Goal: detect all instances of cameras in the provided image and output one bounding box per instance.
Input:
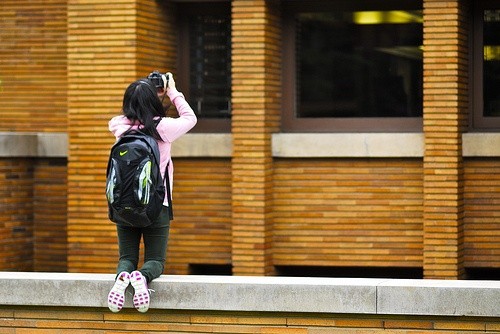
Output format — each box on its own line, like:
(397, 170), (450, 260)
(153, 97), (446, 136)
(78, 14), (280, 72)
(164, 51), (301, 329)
(148, 71), (169, 88)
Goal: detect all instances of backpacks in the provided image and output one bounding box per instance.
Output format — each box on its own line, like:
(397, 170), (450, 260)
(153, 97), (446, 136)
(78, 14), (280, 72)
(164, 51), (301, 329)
(104, 114), (174, 230)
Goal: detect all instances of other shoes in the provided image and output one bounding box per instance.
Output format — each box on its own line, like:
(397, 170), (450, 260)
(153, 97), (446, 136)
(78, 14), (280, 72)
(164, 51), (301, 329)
(129, 270), (155, 313)
(107, 271), (130, 312)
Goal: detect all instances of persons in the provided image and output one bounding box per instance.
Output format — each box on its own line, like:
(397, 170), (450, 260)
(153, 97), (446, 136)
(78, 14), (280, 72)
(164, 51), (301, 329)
(108, 73), (197, 313)
